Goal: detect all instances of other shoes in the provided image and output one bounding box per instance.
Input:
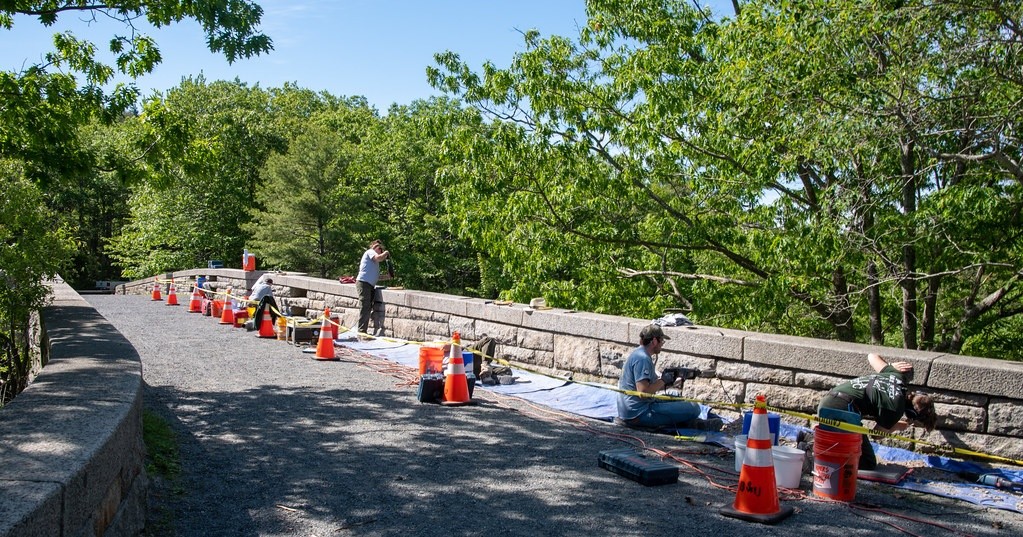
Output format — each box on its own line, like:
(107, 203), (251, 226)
(492, 374), (515, 385)
(358, 335), (369, 341)
(480, 362), (492, 379)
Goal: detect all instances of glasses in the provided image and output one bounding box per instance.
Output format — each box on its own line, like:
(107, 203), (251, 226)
(657, 339), (665, 344)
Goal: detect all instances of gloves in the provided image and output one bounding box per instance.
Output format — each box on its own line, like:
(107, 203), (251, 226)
(662, 367), (679, 386)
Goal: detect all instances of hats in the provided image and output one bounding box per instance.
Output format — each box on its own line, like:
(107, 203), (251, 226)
(639, 324), (671, 340)
(265, 278), (274, 284)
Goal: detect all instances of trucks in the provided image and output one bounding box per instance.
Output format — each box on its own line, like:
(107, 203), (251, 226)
(207, 260), (224, 269)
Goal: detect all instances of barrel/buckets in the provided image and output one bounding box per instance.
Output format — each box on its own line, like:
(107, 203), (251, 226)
(735, 435), (747, 471)
(771, 446), (805, 488)
(420, 347), (444, 374)
(243, 256), (255, 270)
(330, 317), (339, 339)
(812, 425), (862, 501)
(210, 301), (224, 317)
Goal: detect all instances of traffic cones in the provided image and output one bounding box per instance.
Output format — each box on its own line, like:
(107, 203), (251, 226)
(311, 308), (340, 361)
(151, 277), (163, 301)
(164, 277), (180, 306)
(255, 303), (278, 338)
(188, 283), (203, 313)
(719, 396), (795, 525)
(441, 334), (478, 407)
(218, 289), (234, 324)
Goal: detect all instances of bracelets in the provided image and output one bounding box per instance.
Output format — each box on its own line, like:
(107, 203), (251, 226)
(888, 362), (891, 366)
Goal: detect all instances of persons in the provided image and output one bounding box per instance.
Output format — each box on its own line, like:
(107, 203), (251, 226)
(248, 277), (274, 300)
(354, 240), (390, 339)
(796, 353), (938, 472)
(617, 325), (724, 430)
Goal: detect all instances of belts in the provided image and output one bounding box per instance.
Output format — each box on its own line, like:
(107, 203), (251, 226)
(829, 391), (859, 409)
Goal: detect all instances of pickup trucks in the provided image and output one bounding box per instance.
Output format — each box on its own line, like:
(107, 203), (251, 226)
(95, 276), (134, 294)
